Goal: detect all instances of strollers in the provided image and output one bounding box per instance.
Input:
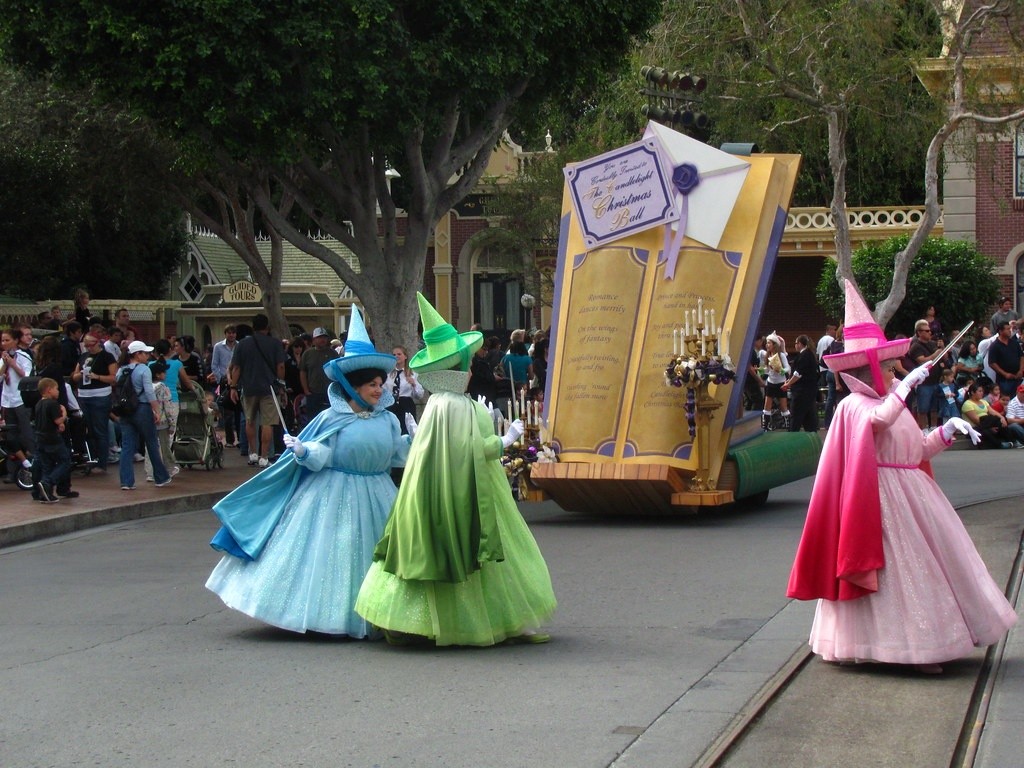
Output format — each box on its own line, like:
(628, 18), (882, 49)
(171, 379), (226, 471)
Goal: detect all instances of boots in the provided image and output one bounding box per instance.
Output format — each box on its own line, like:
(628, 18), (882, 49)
(780, 410), (790, 429)
(761, 409), (775, 433)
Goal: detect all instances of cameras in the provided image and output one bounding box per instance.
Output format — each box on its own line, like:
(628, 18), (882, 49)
(7, 348), (16, 359)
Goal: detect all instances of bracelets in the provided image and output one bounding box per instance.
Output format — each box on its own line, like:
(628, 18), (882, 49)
(230, 385), (238, 389)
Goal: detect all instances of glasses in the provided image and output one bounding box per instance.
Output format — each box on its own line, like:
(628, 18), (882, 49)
(918, 330), (931, 332)
(882, 367), (895, 374)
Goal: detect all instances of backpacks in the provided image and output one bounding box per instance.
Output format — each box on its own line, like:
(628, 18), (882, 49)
(111, 363), (144, 420)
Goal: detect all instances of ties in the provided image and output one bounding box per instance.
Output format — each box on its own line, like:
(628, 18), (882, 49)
(392, 370), (403, 411)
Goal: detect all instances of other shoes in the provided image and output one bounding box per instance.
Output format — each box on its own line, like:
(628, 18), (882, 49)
(1008, 441), (1013, 448)
(1014, 440), (1024, 449)
(914, 664), (943, 674)
(259, 458), (273, 468)
(510, 634), (548, 642)
(248, 453), (259, 465)
(14, 447), (179, 503)
(379, 628), (419, 646)
(225, 443), (235, 448)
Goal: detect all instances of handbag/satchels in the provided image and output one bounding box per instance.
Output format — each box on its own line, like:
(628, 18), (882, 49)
(273, 377), (286, 394)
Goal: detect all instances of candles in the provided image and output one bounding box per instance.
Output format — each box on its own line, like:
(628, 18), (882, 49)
(673, 297), (722, 358)
(477, 387), (538, 446)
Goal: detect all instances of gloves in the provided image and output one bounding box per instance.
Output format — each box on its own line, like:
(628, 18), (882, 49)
(405, 413), (418, 438)
(899, 360), (934, 389)
(942, 417), (982, 445)
(478, 394), (494, 421)
(283, 433), (307, 458)
(502, 419), (525, 448)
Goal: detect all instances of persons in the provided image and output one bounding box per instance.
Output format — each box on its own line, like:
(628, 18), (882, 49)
(204, 304), (417, 640)
(0, 291), (240, 503)
(817, 320), (846, 429)
(226, 313), (375, 468)
(786, 279), (1017, 672)
(743, 331), (821, 432)
(354, 291), (556, 647)
(892, 297), (1024, 448)
(381, 347), (424, 484)
(468, 325), (551, 420)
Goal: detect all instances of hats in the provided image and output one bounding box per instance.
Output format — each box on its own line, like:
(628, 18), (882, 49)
(408, 290), (483, 373)
(1017, 384), (1024, 394)
(322, 303), (397, 383)
(511, 328), (526, 341)
(128, 341), (154, 354)
(330, 339), (344, 356)
(313, 327), (328, 338)
(765, 331), (787, 354)
(823, 278), (911, 372)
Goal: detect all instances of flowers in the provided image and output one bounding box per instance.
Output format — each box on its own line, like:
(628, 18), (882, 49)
(671, 163), (699, 194)
